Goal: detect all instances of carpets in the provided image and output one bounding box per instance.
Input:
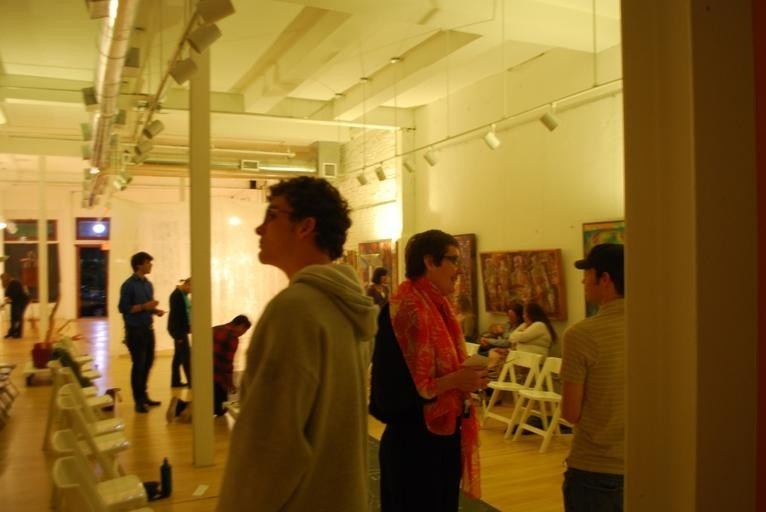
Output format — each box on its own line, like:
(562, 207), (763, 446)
(368, 433), (498, 512)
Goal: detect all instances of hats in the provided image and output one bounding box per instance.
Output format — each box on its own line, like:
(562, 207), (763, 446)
(574, 243), (624, 278)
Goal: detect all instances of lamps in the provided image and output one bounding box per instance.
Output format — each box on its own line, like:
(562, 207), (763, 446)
(353, 101), (562, 189)
(70, 0), (239, 212)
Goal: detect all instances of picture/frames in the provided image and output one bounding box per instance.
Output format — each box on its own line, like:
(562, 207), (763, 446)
(449, 233), (479, 338)
(582, 219), (628, 319)
(478, 248), (567, 322)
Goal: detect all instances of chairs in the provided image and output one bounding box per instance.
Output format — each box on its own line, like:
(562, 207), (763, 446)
(480, 349), (550, 440)
(515, 354), (576, 454)
(463, 341), (489, 418)
(0, 355), (152, 512)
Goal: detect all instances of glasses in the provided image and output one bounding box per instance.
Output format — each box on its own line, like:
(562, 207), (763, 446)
(441, 254), (462, 267)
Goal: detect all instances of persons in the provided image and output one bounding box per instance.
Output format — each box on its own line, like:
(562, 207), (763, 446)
(368, 230), (490, 512)
(0, 272), (31, 339)
(168, 278), (192, 388)
(559, 243), (624, 512)
(369, 268), (389, 310)
(119, 252), (165, 413)
(166, 314), (250, 423)
(214, 176), (381, 511)
(481, 302), (557, 407)
